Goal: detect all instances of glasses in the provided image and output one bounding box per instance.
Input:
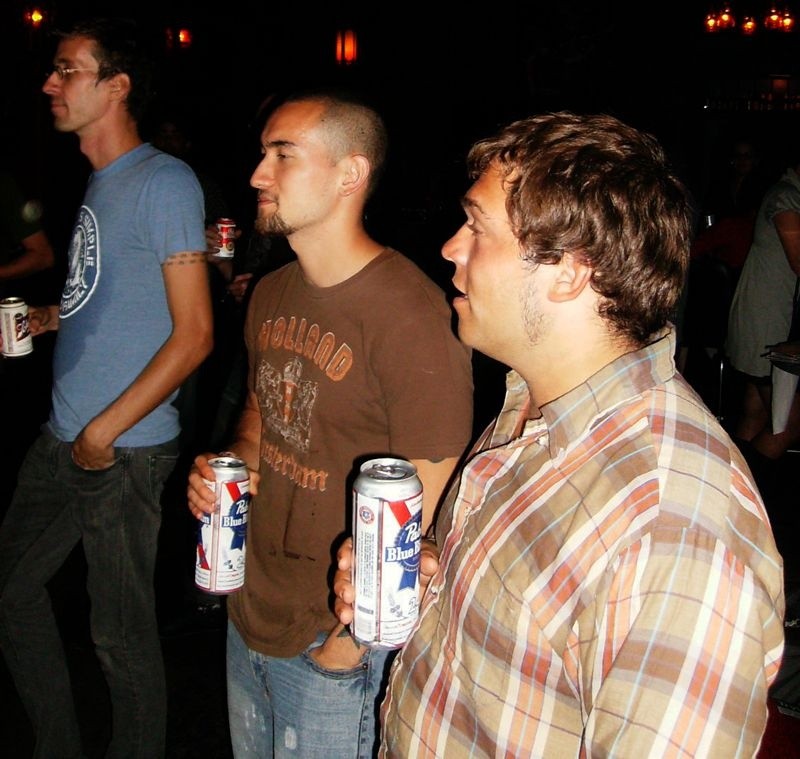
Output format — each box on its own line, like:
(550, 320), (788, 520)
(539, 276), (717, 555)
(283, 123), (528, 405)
(46, 58), (100, 80)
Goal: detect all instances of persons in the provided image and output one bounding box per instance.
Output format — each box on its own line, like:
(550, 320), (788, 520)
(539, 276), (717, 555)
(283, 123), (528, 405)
(187, 97), (474, 757)
(333, 110), (785, 759)
(0, 22), (254, 759)
(725, 151), (800, 490)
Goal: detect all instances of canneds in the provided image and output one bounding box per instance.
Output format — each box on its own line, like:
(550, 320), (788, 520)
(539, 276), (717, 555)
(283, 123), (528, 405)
(212, 218), (237, 259)
(194, 457), (250, 594)
(0, 296), (33, 358)
(350, 458), (427, 649)
(704, 214), (715, 229)
